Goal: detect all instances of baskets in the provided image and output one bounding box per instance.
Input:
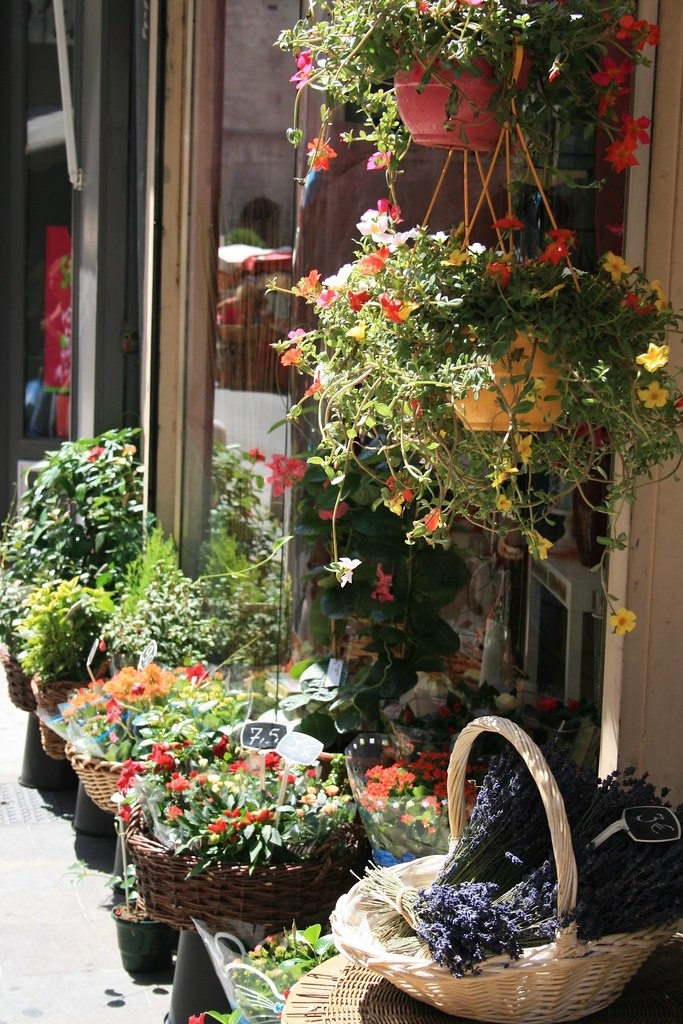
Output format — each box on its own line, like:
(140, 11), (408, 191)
(0, 627), (683, 1024)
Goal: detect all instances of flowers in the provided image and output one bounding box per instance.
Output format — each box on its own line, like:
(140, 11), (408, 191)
(0, 429), (683, 1024)
(273, 0), (661, 261)
(261, 213), (683, 635)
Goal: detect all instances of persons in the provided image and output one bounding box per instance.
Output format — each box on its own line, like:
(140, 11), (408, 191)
(239, 196), (281, 248)
(25, 366), (44, 424)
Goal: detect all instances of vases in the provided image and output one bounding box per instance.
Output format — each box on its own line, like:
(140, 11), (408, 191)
(441, 321), (566, 431)
(111, 835), (135, 892)
(110, 902), (177, 970)
(389, 41), (512, 151)
(163, 927), (234, 1024)
(74, 781), (119, 837)
(18, 713), (81, 792)
